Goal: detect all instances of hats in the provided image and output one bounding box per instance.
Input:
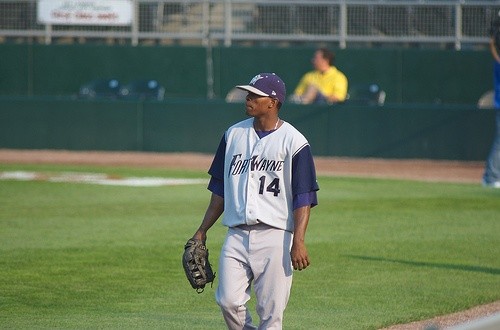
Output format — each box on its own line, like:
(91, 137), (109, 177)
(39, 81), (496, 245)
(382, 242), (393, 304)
(235, 73), (286, 104)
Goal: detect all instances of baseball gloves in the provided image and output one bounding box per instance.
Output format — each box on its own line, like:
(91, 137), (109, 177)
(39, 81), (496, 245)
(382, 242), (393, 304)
(182, 238), (217, 294)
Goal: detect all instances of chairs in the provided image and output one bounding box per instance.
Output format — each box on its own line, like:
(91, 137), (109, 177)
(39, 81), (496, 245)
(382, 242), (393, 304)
(225, 85), (385, 105)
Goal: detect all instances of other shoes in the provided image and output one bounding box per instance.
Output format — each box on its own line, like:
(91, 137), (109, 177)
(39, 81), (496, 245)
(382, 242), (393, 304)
(482, 182), (500, 190)
(289, 94), (301, 105)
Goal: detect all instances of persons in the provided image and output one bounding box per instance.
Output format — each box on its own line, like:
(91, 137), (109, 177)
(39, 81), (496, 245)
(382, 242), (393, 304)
(287, 48), (348, 105)
(182, 72), (318, 330)
(481, 7), (499, 187)
